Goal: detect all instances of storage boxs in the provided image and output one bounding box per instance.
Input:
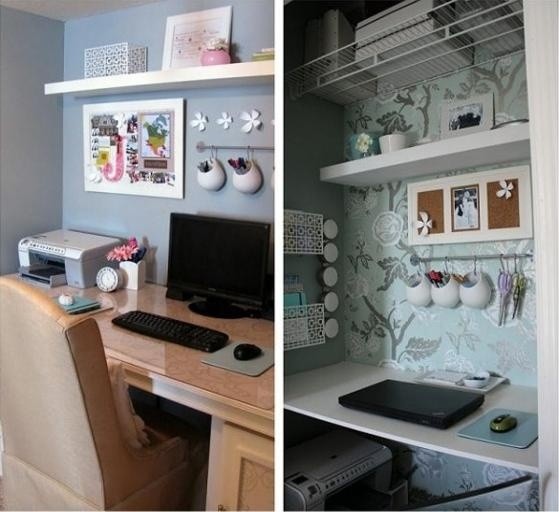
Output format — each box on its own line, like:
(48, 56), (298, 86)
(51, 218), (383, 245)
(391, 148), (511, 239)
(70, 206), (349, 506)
(352, 1), (473, 86)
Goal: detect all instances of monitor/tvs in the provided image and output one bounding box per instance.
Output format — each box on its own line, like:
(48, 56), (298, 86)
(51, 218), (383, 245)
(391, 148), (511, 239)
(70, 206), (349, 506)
(165, 212), (272, 318)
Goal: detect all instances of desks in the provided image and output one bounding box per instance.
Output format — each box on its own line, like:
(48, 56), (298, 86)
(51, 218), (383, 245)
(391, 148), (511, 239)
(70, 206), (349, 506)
(1, 271), (275, 512)
(283, 361), (540, 476)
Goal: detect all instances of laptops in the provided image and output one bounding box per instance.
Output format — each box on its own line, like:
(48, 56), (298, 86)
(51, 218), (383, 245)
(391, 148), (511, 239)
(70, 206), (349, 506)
(337, 379), (486, 430)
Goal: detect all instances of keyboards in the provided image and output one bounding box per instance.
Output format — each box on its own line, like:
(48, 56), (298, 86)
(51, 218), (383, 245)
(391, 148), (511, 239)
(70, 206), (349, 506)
(112, 308), (229, 354)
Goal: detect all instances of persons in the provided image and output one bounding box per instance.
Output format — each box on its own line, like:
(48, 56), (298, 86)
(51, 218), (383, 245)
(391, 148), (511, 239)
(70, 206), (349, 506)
(459, 198), (478, 228)
(455, 189), (477, 226)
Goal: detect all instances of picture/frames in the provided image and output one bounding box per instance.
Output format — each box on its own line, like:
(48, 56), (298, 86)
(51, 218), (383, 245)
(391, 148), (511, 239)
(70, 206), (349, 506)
(440, 93), (494, 139)
(161, 5), (232, 69)
(406, 164), (533, 245)
(81, 97), (186, 199)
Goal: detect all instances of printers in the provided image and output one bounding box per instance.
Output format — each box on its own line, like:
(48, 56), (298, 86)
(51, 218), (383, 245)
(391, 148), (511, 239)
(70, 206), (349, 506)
(17, 227), (119, 290)
(284, 427), (410, 511)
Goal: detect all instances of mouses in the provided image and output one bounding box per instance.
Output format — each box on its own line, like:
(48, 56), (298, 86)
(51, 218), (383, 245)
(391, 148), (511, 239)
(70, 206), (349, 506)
(233, 342), (263, 360)
(488, 413), (518, 432)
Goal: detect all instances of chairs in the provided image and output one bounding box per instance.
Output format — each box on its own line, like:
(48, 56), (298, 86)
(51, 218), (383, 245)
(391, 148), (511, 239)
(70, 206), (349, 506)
(0, 276), (188, 512)
